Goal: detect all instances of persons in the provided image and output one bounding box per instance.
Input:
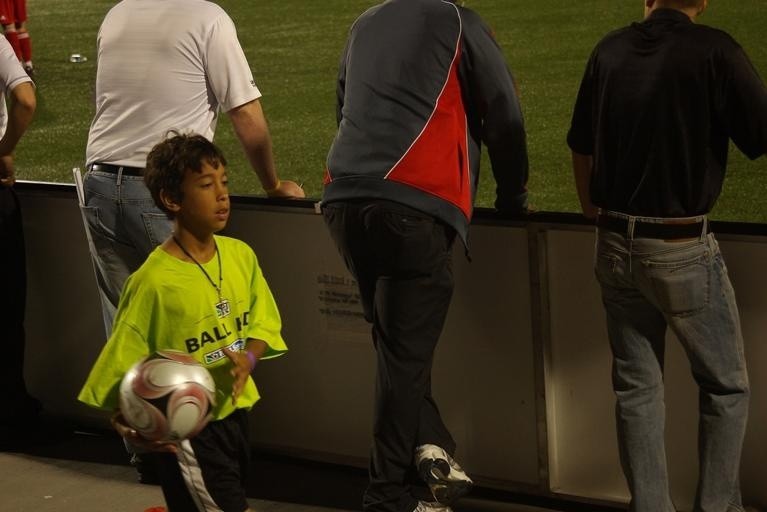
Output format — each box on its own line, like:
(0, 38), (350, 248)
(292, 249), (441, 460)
(565, 1), (767, 512)
(76, 129), (288, 512)
(321, 0), (534, 512)
(0, 0), (33, 73)
(79, 0), (309, 483)
(0, 31), (85, 460)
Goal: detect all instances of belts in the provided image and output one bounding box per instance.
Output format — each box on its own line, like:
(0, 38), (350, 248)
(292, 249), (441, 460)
(595, 213), (710, 240)
(91, 163), (145, 176)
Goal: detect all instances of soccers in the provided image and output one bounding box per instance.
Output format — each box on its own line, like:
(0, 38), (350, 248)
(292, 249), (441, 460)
(120, 348), (216, 442)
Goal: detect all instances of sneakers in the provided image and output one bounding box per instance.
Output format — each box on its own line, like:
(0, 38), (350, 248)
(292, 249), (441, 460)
(413, 498), (453, 512)
(414, 444), (475, 505)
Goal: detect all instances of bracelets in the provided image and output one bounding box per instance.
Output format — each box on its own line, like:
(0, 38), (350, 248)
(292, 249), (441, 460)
(241, 351), (257, 370)
(264, 181), (280, 193)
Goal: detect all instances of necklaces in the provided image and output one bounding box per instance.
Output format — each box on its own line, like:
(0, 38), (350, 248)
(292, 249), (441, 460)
(172, 235), (222, 303)
